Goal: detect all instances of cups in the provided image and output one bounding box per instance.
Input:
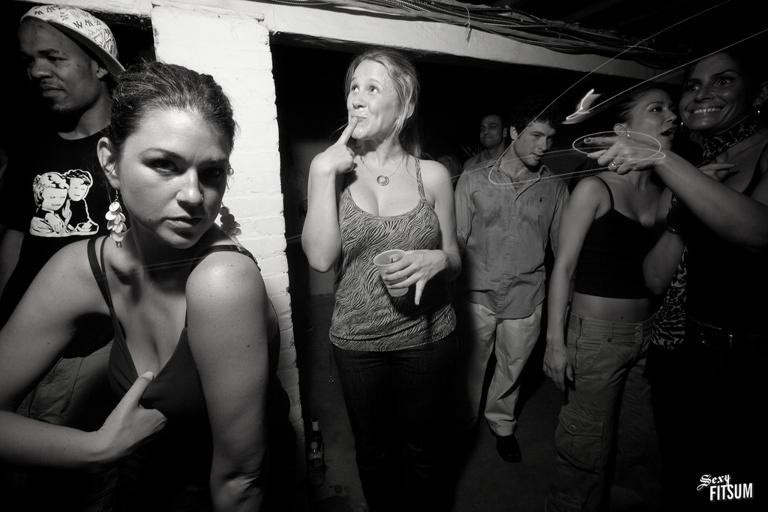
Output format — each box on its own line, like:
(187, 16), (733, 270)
(372, 249), (409, 298)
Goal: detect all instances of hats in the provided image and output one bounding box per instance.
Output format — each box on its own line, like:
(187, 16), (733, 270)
(21, 5), (126, 77)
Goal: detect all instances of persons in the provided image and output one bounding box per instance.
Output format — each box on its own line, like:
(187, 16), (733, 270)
(300, 45), (768, 512)
(29, 169), (100, 237)
(0, 5), (129, 428)
(0, 62), (311, 512)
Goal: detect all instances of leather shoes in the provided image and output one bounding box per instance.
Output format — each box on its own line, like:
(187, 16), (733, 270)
(489, 426), (520, 463)
